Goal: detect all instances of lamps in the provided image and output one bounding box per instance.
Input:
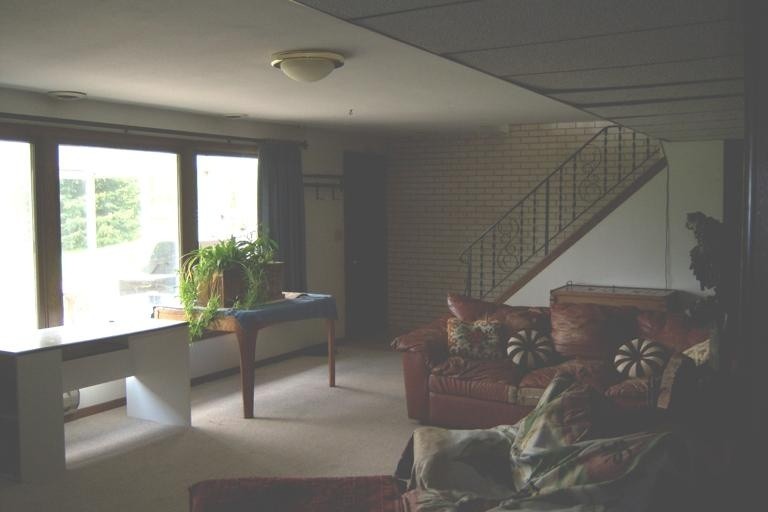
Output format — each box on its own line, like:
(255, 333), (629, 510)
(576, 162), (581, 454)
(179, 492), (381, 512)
(270, 48), (343, 85)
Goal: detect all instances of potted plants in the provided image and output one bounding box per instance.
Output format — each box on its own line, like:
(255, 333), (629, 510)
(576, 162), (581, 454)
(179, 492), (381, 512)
(179, 236), (282, 344)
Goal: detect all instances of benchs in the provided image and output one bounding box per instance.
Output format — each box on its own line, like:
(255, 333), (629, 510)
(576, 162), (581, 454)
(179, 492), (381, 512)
(188, 476), (405, 511)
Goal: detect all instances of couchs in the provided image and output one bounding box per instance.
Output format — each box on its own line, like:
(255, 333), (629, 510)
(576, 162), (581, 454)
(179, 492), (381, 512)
(390, 292), (723, 426)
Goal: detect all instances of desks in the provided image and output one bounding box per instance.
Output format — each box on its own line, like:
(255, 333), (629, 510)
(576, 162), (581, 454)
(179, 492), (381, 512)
(151, 292), (338, 419)
(0, 314), (191, 484)
(551, 286), (676, 309)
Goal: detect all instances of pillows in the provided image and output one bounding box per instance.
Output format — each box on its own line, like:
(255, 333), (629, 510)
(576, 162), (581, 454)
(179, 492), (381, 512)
(446, 311), (711, 408)
